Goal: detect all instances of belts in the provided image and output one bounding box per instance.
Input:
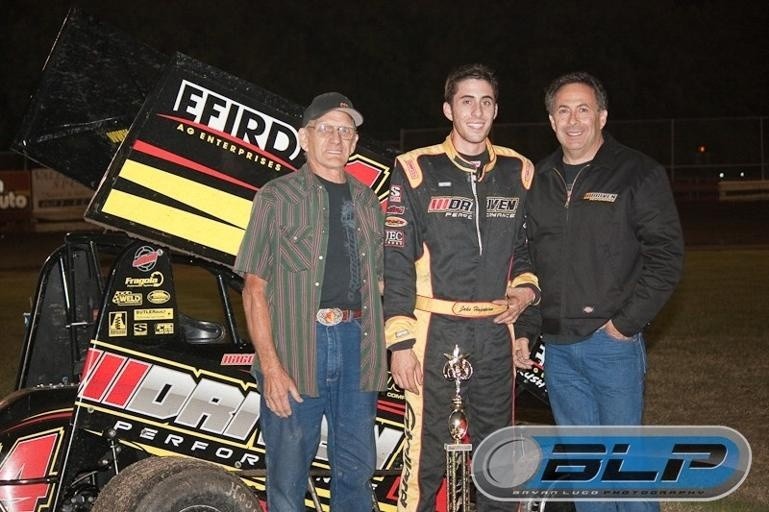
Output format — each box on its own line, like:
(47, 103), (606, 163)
(316, 306), (361, 328)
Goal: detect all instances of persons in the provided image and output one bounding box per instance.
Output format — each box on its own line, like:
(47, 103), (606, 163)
(511, 70), (685, 511)
(233, 92), (389, 512)
(382, 64), (541, 512)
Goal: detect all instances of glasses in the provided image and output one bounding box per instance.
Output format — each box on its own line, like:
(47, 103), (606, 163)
(305, 123), (356, 139)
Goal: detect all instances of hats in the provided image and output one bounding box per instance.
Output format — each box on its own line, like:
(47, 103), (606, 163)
(301, 90), (364, 129)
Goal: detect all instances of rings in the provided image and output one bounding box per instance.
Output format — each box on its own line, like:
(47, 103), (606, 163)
(507, 299), (511, 306)
